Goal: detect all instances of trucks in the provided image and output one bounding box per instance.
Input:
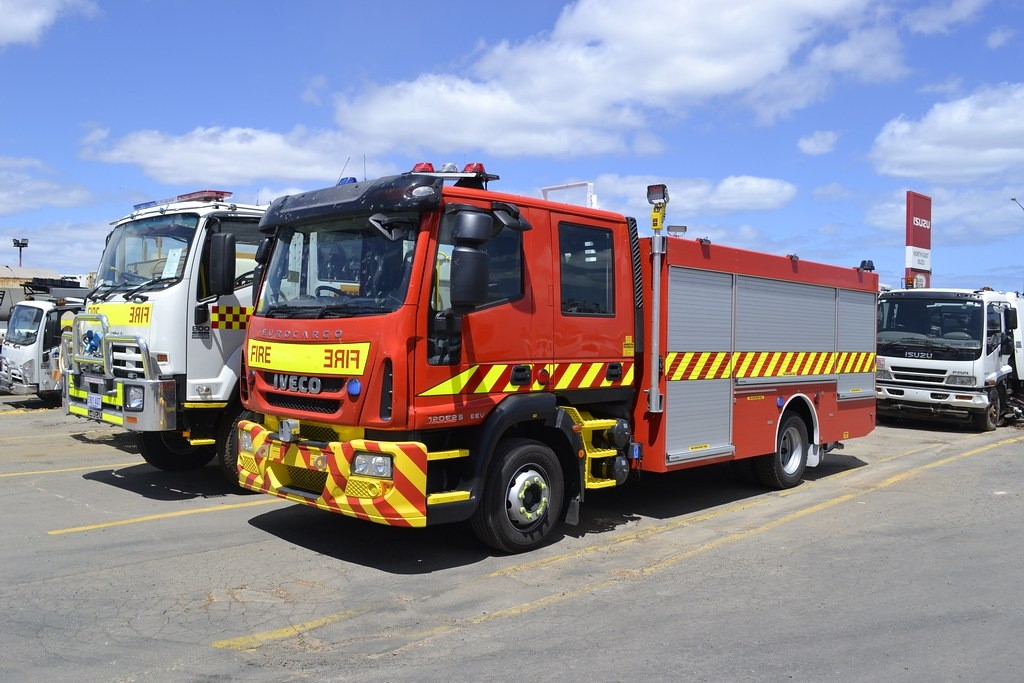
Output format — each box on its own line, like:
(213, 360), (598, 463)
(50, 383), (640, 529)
(875, 287), (1024, 432)
(0, 294), (88, 410)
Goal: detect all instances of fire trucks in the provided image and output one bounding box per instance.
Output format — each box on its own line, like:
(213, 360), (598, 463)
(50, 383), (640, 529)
(236, 161), (878, 552)
(62, 190), (457, 489)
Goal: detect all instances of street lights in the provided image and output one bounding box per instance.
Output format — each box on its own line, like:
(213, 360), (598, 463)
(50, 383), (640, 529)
(13, 238), (29, 267)
